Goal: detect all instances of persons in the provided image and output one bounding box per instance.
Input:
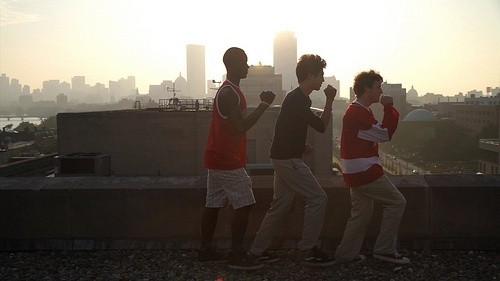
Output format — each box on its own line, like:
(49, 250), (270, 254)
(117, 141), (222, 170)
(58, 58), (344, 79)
(246, 52), (338, 267)
(198, 46), (277, 272)
(333, 70), (411, 265)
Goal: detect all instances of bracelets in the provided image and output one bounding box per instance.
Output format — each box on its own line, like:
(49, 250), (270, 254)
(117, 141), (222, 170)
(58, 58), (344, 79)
(324, 106), (332, 111)
(261, 100), (270, 105)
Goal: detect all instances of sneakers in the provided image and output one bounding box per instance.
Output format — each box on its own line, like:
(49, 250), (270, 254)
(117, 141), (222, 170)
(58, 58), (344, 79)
(373, 250), (411, 264)
(336, 254), (367, 265)
(227, 253), (264, 270)
(246, 252), (281, 263)
(196, 245), (229, 263)
(303, 248), (337, 266)
